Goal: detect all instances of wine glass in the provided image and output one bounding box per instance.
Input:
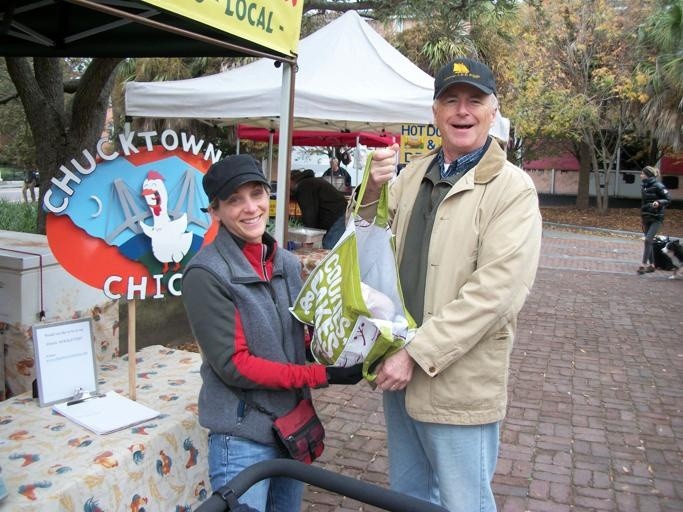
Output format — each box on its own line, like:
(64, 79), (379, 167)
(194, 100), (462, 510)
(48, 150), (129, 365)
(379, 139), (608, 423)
(392, 382), (400, 389)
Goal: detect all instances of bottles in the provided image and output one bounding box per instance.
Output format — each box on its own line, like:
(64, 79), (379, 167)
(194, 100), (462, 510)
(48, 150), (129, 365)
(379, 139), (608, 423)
(642, 165), (656, 177)
(202, 153), (272, 203)
(435, 58), (495, 100)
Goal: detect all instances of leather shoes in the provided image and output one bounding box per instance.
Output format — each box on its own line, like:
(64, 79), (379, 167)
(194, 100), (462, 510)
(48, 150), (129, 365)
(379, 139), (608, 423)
(326, 359), (375, 385)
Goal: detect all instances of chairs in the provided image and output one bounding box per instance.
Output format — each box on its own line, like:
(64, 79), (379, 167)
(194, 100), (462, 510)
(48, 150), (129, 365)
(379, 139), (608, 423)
(637, 266), (656, 273)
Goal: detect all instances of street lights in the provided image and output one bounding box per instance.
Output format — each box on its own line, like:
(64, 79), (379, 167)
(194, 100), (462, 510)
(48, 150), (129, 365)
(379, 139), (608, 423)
(289, 152), (418, 380)
(272, 398), (325, 465)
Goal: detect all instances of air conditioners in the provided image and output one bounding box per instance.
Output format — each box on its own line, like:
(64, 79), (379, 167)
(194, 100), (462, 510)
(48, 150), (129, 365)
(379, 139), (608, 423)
(660, 233), (683, 282)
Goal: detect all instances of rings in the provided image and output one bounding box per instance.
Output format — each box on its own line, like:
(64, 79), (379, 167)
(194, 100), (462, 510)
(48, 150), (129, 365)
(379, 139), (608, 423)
(67, 394), (106, 406)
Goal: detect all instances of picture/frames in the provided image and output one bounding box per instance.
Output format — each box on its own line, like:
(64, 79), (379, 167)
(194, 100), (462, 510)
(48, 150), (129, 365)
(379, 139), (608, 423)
(0, 345), (212, 511)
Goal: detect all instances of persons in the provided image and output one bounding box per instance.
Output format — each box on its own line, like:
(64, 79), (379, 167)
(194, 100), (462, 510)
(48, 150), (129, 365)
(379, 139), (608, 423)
(351, 55), (543, 510)
(320, 156), (351, 187)
(289, 169), (348, 250)
(635, 165), (673, 274)
(178, 151), (385, 510)
